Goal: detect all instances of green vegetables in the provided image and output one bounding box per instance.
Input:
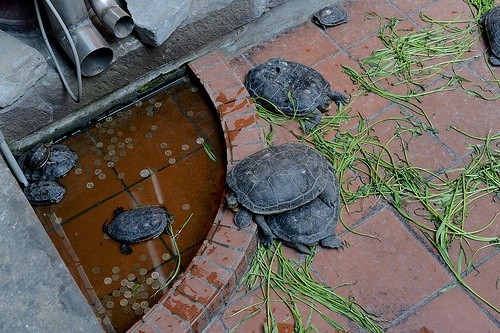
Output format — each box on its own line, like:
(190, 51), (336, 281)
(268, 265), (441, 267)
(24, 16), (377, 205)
(226, 0), (500, 332)
